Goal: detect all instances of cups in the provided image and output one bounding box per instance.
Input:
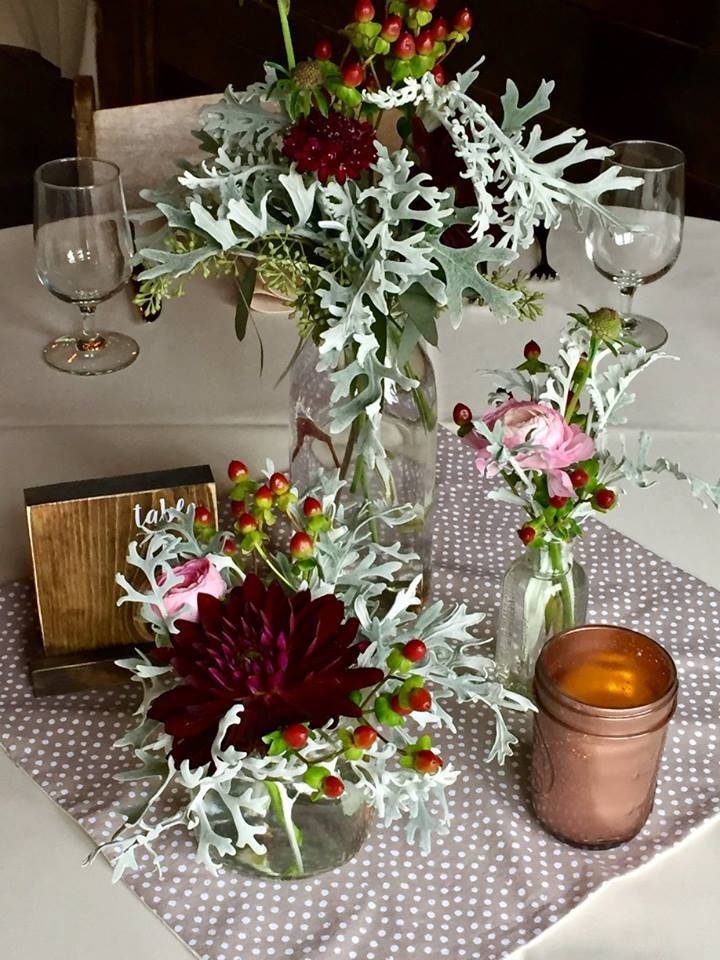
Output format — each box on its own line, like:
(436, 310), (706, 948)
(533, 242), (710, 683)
(530, 624), (679, 851)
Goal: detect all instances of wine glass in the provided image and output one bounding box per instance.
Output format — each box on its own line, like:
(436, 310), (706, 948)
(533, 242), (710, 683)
(584, 141), (684, 353)
(33, 156), (140, 376)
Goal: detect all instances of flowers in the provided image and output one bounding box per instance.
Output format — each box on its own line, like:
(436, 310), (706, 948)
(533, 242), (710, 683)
(82, 460), (539, 882)
(450, 308), (719, 547)
(128, 0), (644, 468)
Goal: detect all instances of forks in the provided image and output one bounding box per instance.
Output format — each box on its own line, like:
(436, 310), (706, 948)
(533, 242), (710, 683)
(530, 219), (558, 280)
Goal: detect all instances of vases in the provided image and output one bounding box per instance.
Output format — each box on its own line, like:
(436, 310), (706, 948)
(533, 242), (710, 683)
(492, 530), (590, 687)
(190, 767), (373, 878)
(290, 319), (436, 605)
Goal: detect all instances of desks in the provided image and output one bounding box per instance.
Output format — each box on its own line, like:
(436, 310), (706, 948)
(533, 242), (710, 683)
(0, 202), (720, 960)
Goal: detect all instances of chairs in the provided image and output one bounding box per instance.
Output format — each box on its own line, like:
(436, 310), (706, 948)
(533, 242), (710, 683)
(72, 86), (297, 214)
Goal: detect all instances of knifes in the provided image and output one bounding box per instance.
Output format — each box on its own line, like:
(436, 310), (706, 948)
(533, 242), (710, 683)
(129, 219), (163, 322)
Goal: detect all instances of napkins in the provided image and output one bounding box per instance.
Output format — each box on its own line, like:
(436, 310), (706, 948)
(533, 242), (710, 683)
(235, 258), (298, 317)
